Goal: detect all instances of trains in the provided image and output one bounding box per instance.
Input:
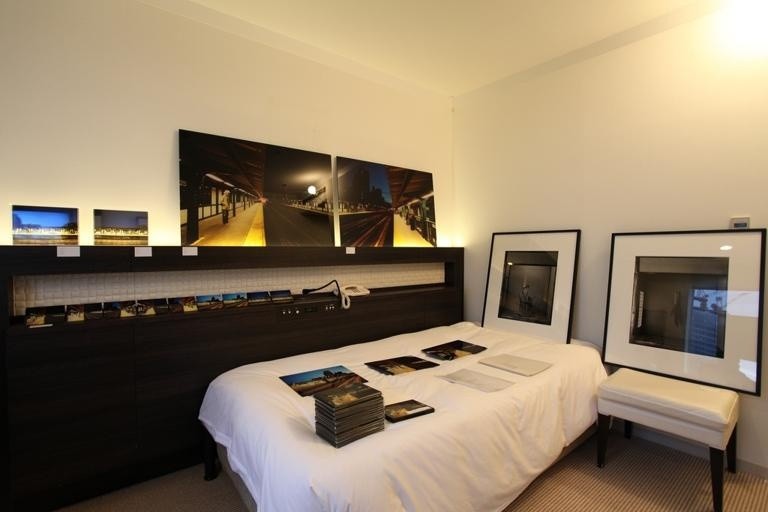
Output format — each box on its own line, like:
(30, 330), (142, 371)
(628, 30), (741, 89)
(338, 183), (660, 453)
(397, 191), (437, 248)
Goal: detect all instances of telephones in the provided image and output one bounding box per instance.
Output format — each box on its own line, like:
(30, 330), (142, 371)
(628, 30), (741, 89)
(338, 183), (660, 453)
(333, 284), (370, 297)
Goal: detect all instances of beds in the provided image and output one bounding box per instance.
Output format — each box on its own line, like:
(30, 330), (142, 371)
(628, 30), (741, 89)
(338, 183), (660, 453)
(197, 320), (608, 512)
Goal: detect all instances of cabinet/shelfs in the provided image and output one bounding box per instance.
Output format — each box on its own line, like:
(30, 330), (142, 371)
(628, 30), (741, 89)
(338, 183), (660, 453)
(0, 246), (465, 512)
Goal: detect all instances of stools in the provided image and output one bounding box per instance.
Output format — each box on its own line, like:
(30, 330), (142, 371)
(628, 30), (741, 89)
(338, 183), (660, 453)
(597, 367), (740, 512)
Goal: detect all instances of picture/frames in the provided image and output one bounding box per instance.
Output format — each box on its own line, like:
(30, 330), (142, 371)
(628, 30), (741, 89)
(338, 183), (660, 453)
(481, 231), (585, 345)
(602, 228), (768, 397)
